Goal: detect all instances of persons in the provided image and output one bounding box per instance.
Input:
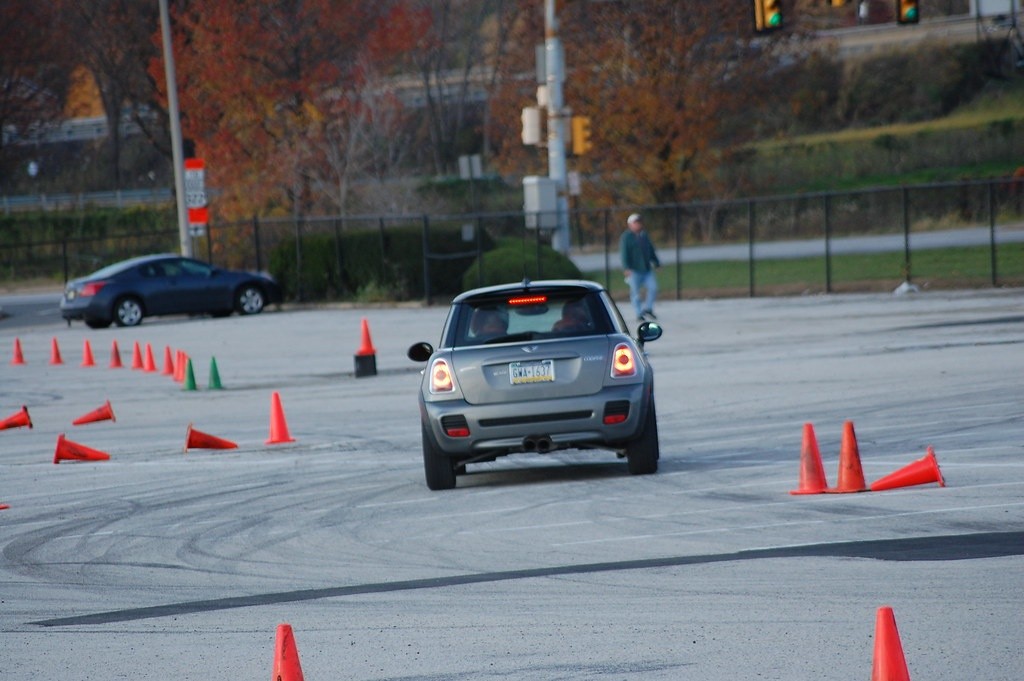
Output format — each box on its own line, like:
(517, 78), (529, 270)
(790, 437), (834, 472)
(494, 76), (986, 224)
(471, 307), (509, 335)
(562, 303), (589, 320)
(620, 213), (661, 321)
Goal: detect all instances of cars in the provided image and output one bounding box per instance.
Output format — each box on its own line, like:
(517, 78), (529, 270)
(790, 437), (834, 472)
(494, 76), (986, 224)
(58, 254), (283, 329)
(406, 278), (666, 490)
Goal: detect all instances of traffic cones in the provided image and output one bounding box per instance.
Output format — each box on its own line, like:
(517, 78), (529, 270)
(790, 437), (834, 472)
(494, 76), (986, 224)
(263, 392), (296, 446)
(788, 422), (829, 494)
(10, 335), (25, 366)
(53, 433), (110, 463)
(272, 624), (304, 681)
(181, 358), (201, 392)
(183, 423), (238, 452)
(71, 401), (117, 425)
(356, 319), (378, 356)
(820, 418), (869, 493)
(49, 338), (188, 383)
(869, 445), (946, 489)
(203, 356), (227, 390)
(871, 606), (912, 681)
(0, 404), (34, 432)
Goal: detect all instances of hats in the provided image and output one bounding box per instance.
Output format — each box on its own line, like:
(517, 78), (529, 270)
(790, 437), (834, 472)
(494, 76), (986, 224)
(627, 214), (643, 223)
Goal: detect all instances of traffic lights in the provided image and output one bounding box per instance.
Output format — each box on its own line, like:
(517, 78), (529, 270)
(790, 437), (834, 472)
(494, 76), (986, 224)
(755, 1), (781, 33)
(575, 115), (594, 156)
(900, 0), (917, 20)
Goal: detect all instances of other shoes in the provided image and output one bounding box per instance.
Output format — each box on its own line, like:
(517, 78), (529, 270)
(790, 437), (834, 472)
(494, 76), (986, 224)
(636, 316), (646, 323)
(642, 309), (656, 319)
